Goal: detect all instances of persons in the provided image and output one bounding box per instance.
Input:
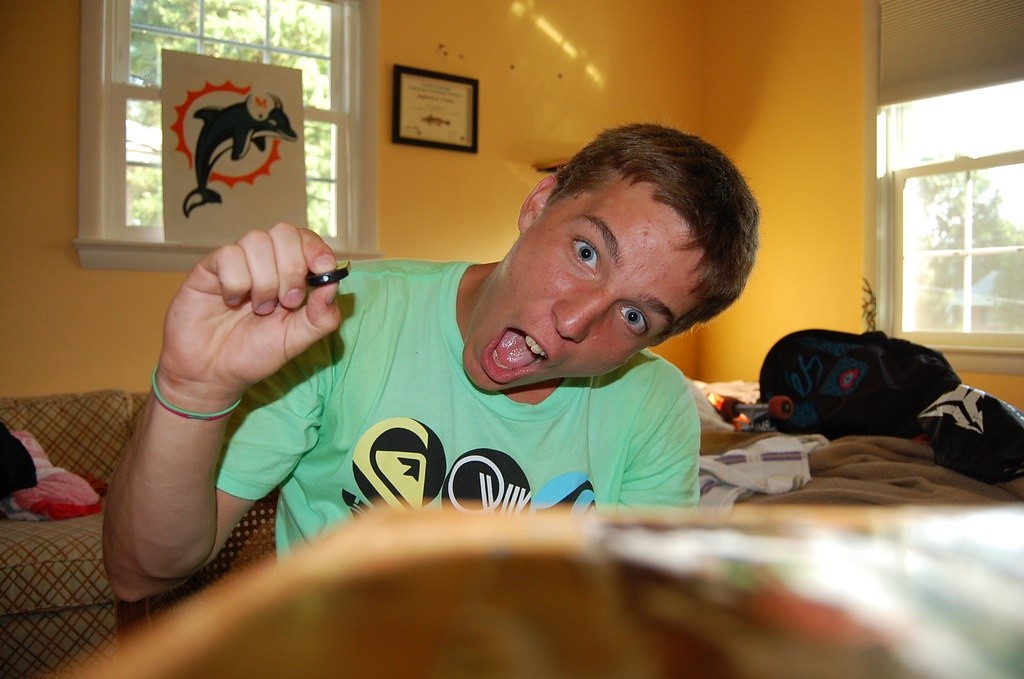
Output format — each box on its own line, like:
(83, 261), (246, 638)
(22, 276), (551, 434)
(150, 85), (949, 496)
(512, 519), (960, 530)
(100, 122), (758, 603)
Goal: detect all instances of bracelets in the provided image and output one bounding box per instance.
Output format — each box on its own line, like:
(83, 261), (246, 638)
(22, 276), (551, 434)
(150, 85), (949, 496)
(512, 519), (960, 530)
(152, 363), (242, 421)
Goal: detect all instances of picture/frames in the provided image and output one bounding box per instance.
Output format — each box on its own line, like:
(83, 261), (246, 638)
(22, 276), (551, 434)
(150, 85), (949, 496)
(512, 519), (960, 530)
(392, 64), (479, 154)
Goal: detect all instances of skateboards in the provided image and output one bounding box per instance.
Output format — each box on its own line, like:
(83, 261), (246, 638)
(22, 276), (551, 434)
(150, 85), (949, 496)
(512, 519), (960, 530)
(699, 396), (796, 456)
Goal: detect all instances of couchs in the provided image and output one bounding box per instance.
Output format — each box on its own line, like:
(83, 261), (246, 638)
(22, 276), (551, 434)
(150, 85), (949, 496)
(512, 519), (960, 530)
(0, 390), (151, 679)
